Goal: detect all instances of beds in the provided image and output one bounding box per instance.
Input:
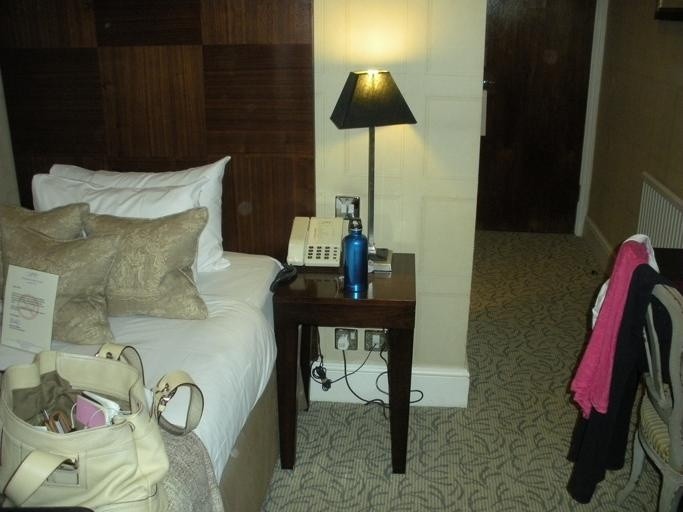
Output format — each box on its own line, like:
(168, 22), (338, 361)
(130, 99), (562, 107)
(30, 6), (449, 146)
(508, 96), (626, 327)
(0, 250), (308, 511)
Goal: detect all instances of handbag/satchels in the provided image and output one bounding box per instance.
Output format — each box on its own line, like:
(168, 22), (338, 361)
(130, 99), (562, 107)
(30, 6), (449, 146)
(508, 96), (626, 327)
(0, 339), (206, 510)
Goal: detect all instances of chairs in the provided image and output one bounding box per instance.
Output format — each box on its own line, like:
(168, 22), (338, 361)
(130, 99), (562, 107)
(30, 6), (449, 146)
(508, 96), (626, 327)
(607, 283), (683, 511)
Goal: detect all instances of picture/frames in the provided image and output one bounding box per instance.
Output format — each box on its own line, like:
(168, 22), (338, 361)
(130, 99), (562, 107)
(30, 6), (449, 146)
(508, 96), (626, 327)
(652, 0), (683, 23)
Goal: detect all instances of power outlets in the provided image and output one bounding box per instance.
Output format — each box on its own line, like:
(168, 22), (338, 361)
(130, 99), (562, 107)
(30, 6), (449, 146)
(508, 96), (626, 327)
(363, 329), (387, 352)
(334, 328), (358, 351)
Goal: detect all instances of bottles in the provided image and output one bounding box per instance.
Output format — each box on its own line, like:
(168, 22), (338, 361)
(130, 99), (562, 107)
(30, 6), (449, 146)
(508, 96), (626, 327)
(343, 219), (368, 291)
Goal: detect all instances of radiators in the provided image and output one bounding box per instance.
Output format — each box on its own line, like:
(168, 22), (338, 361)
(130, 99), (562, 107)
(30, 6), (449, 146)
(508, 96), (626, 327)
(636, 168), (683, 250)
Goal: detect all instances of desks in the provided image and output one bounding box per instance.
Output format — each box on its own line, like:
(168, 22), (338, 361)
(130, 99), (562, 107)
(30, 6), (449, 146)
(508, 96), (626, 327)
(269, 250), (419, 468)
(649, 245), (683, 381)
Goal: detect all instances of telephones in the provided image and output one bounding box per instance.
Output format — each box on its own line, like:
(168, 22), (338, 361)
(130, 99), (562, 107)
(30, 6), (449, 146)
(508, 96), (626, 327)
(286, 215), (344, 268)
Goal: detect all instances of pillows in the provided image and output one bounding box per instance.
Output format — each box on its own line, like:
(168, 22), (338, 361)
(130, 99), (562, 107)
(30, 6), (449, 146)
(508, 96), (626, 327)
(0, 196), (88, 242)
(77, 201), (210, 323)
(0, 219), (124, 347)
(27, 171), (207, 281)
(47, 151), (234, 274)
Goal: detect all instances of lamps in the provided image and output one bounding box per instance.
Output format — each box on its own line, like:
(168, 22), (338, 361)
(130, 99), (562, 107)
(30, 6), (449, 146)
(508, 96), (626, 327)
(327, 69), (418, 273)
